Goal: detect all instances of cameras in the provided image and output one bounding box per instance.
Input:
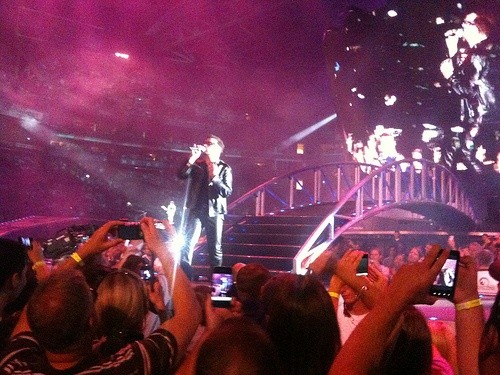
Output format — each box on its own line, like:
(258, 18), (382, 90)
(140, 266), (155, 282)
(18, 236), (33, 249)
(117, 222), (144, 240)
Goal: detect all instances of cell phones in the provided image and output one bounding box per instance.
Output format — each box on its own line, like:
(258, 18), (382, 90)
(430, 249), (460, 299)
(356, 253), (369, 276)
(212, 267), (234, 307)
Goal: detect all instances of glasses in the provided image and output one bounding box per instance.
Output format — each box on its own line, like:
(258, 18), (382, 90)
(462, 19), (475, 26)
(203, 142), (216, 146)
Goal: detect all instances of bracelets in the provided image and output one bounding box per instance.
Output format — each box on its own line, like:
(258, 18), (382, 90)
(187, 160), (192, 166)
(70, 253), (86, 267)
(455, 298), (482, 312)
(354, 285), (367, 300)
(328, 291), (340, 299)
(32, 260), (45, 270)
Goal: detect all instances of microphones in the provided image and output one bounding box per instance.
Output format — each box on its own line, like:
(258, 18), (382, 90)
(189, 145), (206, 152)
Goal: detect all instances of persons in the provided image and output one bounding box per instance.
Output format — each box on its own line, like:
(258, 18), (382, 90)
(0, 214), (500, 375)
(439, 10), (498, 177)
(177, 134), (233, 281)
